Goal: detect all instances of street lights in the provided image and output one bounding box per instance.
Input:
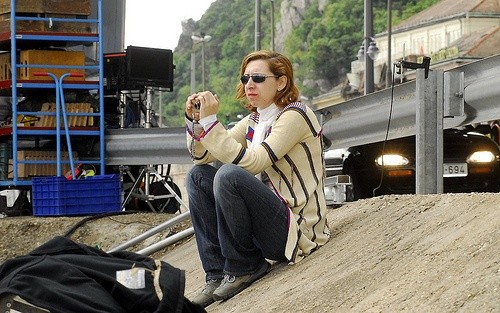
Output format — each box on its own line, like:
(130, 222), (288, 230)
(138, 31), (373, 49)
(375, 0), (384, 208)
(191, 34), (212, 92)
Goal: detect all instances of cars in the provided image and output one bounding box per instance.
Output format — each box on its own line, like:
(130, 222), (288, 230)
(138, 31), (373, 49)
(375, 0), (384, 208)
(340, 126), (500, 197)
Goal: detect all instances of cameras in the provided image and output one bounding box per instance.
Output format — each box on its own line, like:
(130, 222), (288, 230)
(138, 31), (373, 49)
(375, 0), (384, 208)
(195, 90), (215, 109)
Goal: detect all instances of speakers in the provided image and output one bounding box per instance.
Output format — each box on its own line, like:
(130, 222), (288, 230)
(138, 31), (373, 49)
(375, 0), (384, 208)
(123, 46), (174, 88)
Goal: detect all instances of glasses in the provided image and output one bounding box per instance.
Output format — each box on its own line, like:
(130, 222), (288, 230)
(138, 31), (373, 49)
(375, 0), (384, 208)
(239, 73), (279, 83)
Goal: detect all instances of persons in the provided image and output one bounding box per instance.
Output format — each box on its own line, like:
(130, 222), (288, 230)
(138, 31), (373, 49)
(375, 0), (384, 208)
(184, 50), (330, 310)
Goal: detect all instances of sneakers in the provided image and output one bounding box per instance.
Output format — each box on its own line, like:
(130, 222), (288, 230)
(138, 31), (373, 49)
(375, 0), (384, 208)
(193, 277), (222, 307)
(213, 260), (273, 301)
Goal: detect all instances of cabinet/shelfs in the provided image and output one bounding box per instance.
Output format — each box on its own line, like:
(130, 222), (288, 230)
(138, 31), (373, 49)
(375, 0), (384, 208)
(0, 0), (105, 185)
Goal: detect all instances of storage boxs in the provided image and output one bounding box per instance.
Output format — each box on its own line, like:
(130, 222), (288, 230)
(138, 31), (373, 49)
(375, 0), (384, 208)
(32, 174), (123, 216)
(0, 0), (94, 177)
